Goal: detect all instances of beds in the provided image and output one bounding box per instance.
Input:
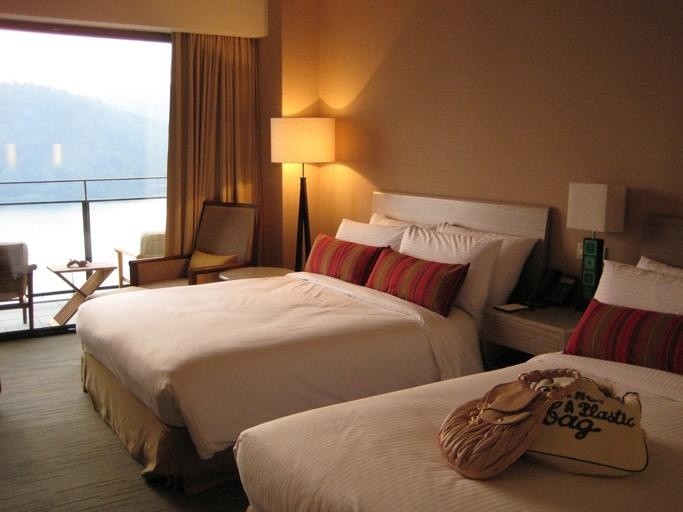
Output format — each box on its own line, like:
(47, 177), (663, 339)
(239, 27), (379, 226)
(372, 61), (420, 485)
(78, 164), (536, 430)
(72, 189), (555, 484)
(230, 211), (681, 512)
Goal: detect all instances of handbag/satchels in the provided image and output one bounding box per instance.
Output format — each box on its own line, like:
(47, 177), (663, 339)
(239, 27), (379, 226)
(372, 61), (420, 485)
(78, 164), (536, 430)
(523, 376), (650, 480)
(436, 368), (583, 480)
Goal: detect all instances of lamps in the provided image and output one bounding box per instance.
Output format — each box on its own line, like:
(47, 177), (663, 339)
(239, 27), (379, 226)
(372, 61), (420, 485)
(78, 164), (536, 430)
(267, 115), (337, 273)
(564, 179), (627, 311)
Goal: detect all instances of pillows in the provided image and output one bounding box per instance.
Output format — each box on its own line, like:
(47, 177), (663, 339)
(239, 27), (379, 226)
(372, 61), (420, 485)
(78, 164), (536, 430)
(563, 252), (681, 375)
(302, 211), (541, 322)
(187, 249), (237, 273)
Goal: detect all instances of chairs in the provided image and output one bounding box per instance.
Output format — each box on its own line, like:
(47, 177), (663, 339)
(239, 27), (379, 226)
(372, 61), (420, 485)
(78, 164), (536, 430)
(113, 232), (167, 289)
(0, 240), (39, 332)
(126, 198), (261, 289)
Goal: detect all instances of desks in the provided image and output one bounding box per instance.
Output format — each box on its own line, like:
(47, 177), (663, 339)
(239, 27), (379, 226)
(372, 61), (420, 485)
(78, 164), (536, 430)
(45, 260), (119, 327)
(216, 264), (298, 282)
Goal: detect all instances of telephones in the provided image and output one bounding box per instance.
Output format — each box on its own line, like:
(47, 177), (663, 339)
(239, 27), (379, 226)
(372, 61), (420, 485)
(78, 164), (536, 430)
(532, 269), (579, 307)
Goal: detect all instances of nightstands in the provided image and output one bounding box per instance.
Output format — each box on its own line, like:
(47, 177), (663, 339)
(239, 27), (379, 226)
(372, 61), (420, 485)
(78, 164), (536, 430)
(478, 303), (585, 357)
